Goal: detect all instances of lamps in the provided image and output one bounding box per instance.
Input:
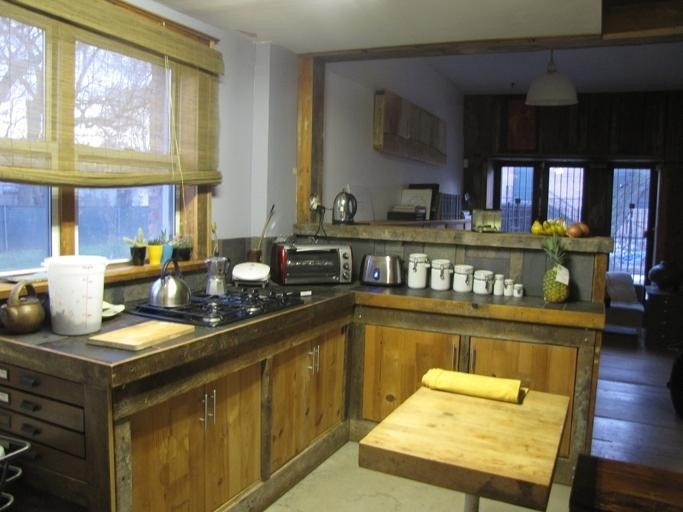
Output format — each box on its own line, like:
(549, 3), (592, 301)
(524, 49), (578, 107)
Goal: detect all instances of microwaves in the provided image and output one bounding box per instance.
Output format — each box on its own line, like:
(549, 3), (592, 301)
(270, 240), (354, 286)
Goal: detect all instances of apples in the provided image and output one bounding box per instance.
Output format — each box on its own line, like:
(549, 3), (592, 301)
(567, 223), (589, 237)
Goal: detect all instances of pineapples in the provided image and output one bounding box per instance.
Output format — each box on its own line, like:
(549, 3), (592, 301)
(543, 266), (569, 302)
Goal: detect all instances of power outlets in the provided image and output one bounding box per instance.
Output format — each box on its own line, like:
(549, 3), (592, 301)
(310, 196), (322, 210)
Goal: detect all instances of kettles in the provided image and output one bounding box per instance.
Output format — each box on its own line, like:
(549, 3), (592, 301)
(329, 189), (359, 225)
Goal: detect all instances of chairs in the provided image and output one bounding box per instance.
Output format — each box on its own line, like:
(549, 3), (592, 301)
(607, 285), (646, 310)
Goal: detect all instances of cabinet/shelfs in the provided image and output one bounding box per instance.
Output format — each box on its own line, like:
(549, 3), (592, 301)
(269, 310), (352, 506)
(646, 280), (683, 353)
(462, 310), (600, 458)
(110, 360), (267, 512)
(372, 217), (473, 231)
(350, 307), (461, 442)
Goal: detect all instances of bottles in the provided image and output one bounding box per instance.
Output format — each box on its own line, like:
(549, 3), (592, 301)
(408, 253), (524, 298)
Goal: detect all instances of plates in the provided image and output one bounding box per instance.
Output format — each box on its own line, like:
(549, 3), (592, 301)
(232, 260), (271, 285)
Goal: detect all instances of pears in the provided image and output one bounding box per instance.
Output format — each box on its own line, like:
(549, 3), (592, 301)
(532, 221), (542, 234)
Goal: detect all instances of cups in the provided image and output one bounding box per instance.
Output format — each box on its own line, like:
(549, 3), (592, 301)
(129, 244), (191, 267)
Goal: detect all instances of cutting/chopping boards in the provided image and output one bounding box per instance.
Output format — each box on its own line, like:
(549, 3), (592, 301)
(86, 315), (196, 351)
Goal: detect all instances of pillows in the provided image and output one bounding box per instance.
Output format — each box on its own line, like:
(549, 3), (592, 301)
(608, 271), (639, 301)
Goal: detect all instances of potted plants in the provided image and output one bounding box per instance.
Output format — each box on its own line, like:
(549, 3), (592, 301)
(149, 237), (167, 263)
(160, 227), (175, 264)
(172, 235), (193, 264)
(121, 226), (146, 266)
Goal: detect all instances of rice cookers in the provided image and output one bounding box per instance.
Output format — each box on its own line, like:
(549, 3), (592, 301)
(358, 253), (403, 288)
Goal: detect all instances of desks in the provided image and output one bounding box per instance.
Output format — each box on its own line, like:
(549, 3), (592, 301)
(359, 382), (572, 512)
(604, 309), (643, 354)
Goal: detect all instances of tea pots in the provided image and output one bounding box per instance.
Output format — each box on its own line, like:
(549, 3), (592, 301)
(150, 257), (192, 308)
(204, 255), (231, 297)
(1, 279), (49, 336)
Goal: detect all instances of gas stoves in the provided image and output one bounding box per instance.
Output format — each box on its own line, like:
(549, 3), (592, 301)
(121, 283), (305, 329)
